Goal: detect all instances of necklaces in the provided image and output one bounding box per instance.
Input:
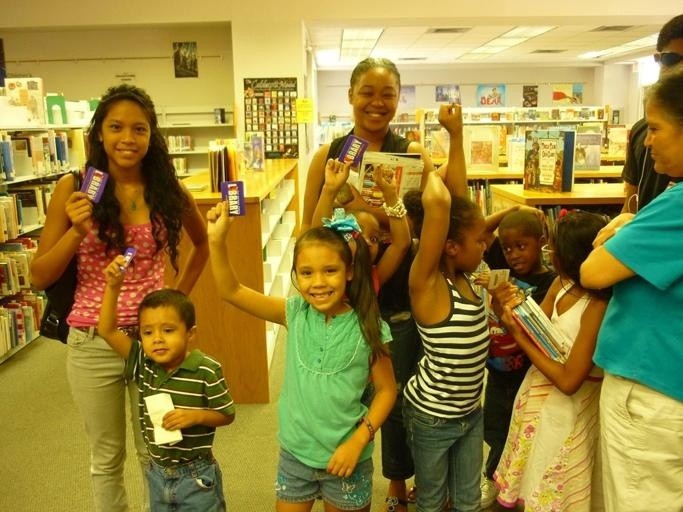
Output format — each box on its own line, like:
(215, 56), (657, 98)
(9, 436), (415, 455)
(114, 177), (145, 212)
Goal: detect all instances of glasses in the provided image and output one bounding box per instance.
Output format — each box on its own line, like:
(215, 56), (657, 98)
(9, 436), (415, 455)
(654, 53), (683, 65)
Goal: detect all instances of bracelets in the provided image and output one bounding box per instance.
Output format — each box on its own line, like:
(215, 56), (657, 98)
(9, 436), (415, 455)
(382, 201), (407, 217)
(354, 415), (376, 443)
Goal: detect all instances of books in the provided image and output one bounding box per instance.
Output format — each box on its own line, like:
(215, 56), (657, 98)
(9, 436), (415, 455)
(0, 75), (101, 360)
(160, 131), (267, 195)
(510, 291), (575, 370)
(321, 122), (353, 144)
(390, 108), (627, 215)
(335, 145), (425, 246)
(358, 151), (423, 208)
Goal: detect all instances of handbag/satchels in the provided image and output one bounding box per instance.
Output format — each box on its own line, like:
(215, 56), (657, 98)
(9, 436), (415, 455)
(39, 253), (76, 343)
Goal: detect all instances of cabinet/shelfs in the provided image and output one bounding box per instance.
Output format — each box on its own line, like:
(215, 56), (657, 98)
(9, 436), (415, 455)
(165, 159), (300, 404)
(0, 125), (88, 366)
(159, 123), (233, 178)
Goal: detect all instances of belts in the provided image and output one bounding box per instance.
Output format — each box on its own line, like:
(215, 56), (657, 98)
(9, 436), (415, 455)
(80, 325), (139, 336)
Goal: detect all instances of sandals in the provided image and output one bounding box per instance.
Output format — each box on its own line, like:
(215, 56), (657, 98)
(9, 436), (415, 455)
(385, 485), (416, 512)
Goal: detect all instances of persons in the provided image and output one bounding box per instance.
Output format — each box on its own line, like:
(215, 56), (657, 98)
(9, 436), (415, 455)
(579, 62), (683, 512)
(96, 253), (236, 512)
(28, 84), (209, 512)
(401, 102), (492, 511)
(204, 200), (397, 512)
(478, 202), (555, 510)
(299, 56), (434, 512)
(309, 157), (411, 311)
(491, 208), (611, 512)
(617, 11), (683, 216)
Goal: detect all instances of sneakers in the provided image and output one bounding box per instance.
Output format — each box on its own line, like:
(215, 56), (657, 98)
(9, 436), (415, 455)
(481, 478), (499, 508)
